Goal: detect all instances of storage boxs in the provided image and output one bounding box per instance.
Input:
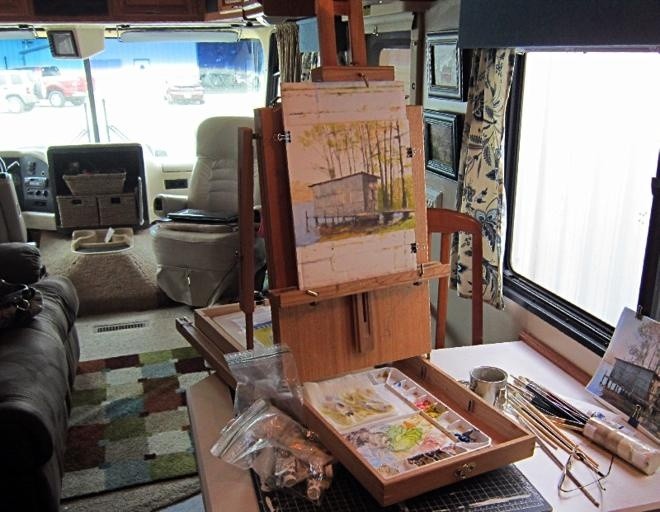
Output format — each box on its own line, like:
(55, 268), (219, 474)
(55, 170), (137, 227)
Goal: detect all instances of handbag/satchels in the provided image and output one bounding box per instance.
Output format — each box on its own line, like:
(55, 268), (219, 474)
(0, 276), (43, 321)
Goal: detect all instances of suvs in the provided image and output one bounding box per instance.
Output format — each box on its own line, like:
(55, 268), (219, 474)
(0, 65), (95, 114)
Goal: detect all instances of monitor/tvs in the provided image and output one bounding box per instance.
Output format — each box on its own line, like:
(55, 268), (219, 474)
(43, 29), (105, 60)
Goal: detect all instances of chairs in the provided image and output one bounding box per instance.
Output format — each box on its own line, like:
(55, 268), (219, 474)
(149, 116), (266, 309)
(425, 204), (484, 350)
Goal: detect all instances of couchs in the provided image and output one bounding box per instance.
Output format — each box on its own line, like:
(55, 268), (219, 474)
(1, 242), (81, 512)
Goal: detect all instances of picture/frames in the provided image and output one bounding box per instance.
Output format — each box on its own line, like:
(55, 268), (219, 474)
(422, 107), (460, 181)
(426, 26), (467, 102)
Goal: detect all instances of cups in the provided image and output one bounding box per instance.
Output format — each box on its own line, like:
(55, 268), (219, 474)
(470, 366), (508, 412)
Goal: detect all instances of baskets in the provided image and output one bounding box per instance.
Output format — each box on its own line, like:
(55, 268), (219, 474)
(61, 168), (127, 196)
(55, 194), (99, 229)
(98, 192), (139, 225)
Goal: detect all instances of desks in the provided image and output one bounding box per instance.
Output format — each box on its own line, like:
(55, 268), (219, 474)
(184, 341), (660, 512)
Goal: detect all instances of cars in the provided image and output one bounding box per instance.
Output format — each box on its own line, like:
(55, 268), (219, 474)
(163, 75), (205, 105)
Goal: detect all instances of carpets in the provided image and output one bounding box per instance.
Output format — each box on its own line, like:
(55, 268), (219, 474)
(57, 347), (219, 510)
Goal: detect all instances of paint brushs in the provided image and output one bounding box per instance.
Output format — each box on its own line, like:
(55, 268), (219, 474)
(505, 375), (604, 507)
